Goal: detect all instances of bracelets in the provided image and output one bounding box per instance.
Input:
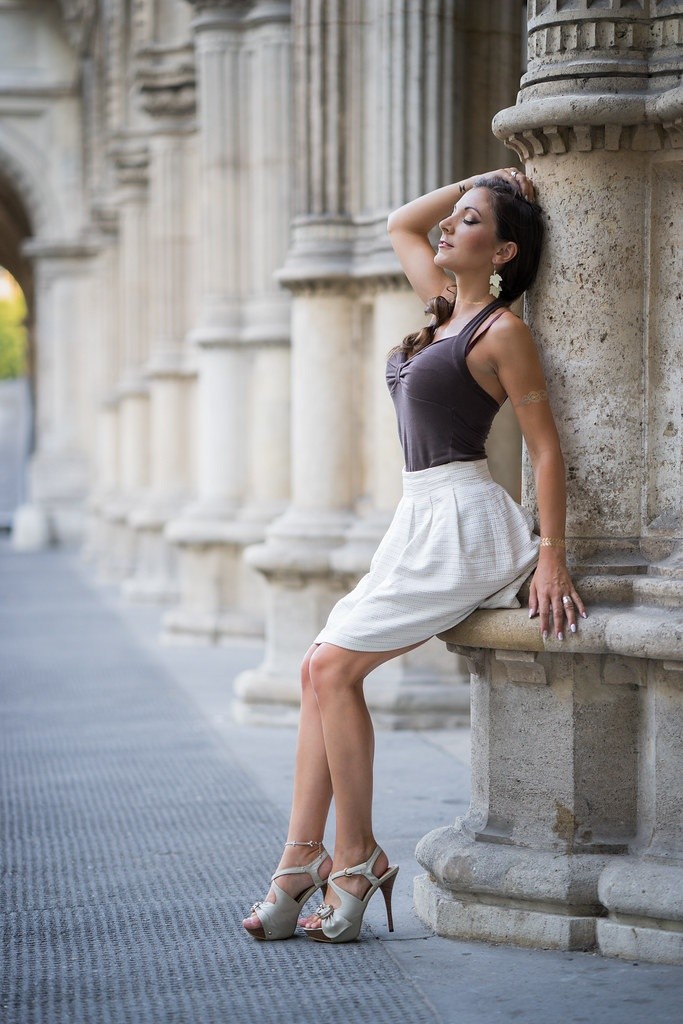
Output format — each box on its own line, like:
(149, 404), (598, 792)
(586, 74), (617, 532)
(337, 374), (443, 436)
(539, 537), (566, 548)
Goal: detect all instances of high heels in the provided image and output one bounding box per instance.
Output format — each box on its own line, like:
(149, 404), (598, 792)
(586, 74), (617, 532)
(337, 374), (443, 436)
(245, 849), (331, 940)
(303, 845), (399, 944)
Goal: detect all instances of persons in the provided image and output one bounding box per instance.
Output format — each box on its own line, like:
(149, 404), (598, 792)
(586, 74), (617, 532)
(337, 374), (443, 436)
(242, 167), (587, 943)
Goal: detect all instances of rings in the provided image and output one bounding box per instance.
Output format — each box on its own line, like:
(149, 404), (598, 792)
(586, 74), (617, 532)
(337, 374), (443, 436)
(510, 170), (518, 178)
(561, 596), (573, 604)
